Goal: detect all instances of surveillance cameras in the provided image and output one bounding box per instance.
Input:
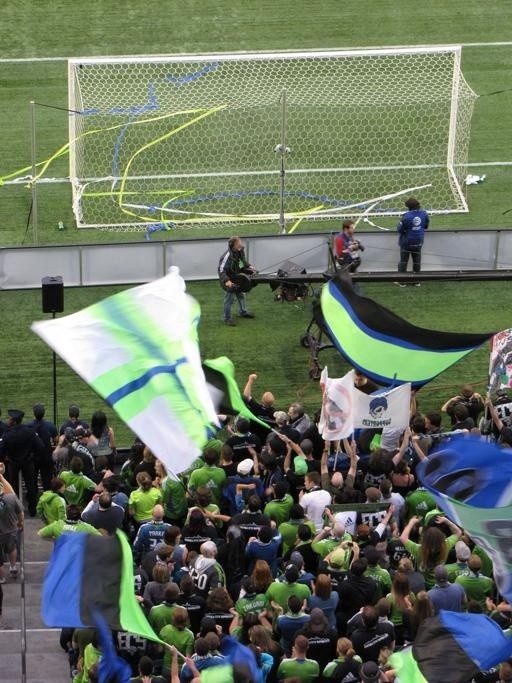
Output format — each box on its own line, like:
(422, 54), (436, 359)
(273, 143), (282, 153)
(285, 146), (291, 155)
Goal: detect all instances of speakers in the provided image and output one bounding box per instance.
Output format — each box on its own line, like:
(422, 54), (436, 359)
(40, 275), (67, 315)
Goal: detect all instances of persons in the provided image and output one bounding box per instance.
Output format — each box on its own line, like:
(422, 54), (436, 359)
(1, 368), (512, 683)
(218, 236), (256, 326)
(393, 198), (429, 287)
(333, 219), (361, 273)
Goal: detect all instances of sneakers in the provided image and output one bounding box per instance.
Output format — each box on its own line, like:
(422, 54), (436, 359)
(0, 570), (18, 583)
(395, 282), (421, 287)
(240, 311), (255, 318)
(224, 320), (235, 326)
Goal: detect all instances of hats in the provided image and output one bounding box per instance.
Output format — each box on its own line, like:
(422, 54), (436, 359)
(290, 551), (302, 571)
(294, 456), (308, 476)
(69, 404), (80, 418)
(237, 459), (252, 476)
(455, 541), (470, 562)
(273, 410), (288, 422)
(330, 549), (344, 567)
(359, 661), (381, 682)
(7, 409), (25, 420)
(33, 404), (45, 419)
(310, 608), (325, 631)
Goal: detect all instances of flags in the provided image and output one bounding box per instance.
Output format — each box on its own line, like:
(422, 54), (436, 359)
(490, 326), (512, 392)
(311, 274), (490, 389)
(22, 265), (221, 471)
